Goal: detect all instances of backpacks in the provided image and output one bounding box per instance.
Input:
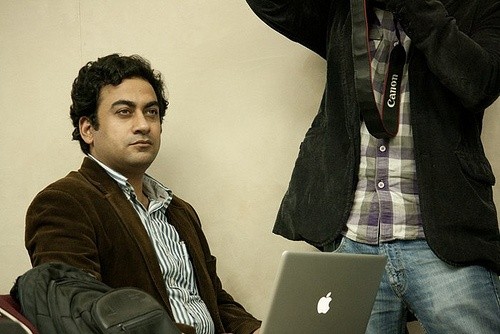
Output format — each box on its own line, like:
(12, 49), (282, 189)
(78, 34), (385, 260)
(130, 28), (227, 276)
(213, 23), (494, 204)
(10, 261), (183, 334)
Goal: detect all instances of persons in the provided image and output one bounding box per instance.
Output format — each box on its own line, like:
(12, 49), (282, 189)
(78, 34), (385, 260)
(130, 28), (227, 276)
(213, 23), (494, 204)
(245, 0), (499, 334)
(25, 54), (262, 334)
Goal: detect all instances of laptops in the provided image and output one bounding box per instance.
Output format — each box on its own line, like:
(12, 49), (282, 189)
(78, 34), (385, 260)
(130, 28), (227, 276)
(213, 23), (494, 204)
(259, 250), (389, 334)
